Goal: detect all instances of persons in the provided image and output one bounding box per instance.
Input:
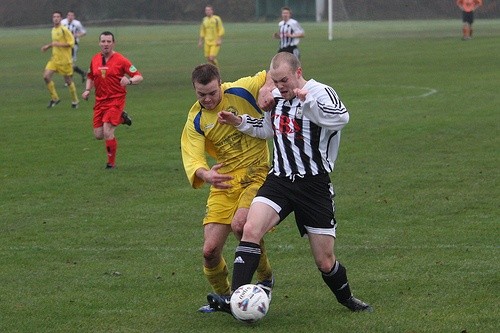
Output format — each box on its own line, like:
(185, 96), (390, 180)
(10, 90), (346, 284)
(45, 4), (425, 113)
(60, 10), (89, 87)
(198, 5), (225, 85)
(181, 64), (277, 312)
(41, 11), (80, 109)
(457, 0), (483, 40)
(82, 31), (143, 169)
(274, 6), (304, 63)
(207, 52), (369, 313)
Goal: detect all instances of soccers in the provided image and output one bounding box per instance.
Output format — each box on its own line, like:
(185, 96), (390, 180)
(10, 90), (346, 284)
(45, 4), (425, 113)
(230, 284), (270, 322)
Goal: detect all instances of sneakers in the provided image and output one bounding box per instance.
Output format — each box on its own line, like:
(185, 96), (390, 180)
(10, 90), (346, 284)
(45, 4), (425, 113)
(121, 112), (132, 126)
(82, 72), (88, 85)
(207, 294), (238, 321)
(71, 100), (80, 109)
(255, 274), (275, 304)
(48, 98), (60, 108)
(106, 163), (112, 168)
(198, 304), (215, 313)
(341, 295), (374, 313)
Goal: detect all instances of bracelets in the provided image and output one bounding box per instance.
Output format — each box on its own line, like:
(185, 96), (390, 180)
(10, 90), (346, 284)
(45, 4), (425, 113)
(85, 88), (91, 91)
(129, 79), (132, 85)
